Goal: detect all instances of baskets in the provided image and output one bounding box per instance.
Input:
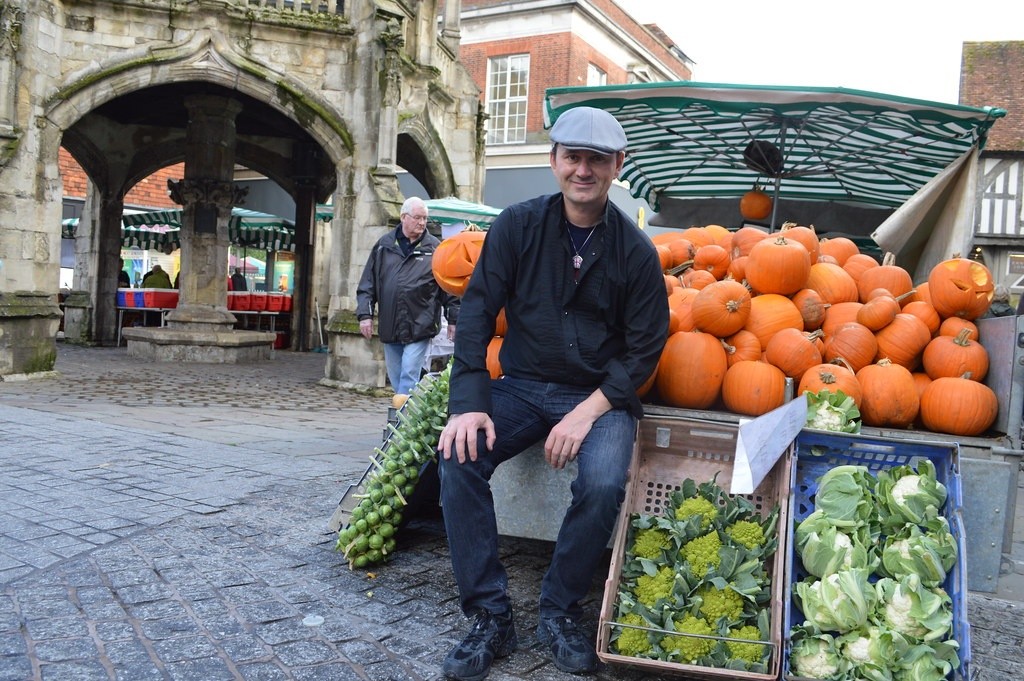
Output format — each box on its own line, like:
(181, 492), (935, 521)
(781, 423), (970, 681)
(596, 415), (794, 679)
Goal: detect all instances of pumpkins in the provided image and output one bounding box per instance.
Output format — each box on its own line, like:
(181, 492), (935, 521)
(653, 224), (999, 435)
(739, 190), (771, 219)
(486, 306), (507, 379)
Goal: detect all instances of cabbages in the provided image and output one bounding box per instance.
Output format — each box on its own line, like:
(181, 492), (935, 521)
(335, 381), (451, 568)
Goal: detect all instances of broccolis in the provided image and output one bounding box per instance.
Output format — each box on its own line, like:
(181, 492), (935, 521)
(802, 389), (862, 437)
(790, 457), (960, 681)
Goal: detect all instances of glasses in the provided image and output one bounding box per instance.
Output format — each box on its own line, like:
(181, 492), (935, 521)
(404, 212), (428, 222)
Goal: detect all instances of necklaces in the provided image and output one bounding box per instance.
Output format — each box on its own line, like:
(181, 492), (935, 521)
(565, 220), (599, 268)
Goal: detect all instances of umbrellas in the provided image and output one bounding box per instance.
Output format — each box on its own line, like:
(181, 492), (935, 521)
(315, 196), (504, 229)
(61, 207), (295, 256)
(542, 80), (1008, 288)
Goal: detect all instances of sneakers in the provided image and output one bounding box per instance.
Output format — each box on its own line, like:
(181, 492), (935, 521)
(442, 603), (518, 681)
(535, 614), (599, 673)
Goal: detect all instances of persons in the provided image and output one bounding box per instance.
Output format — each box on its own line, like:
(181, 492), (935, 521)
(227, 267), (247, 291)
(355, 197), (461, 395)
(134, 265), (180, 290)
(117, 257), (130, 288)
(437, 106), (671, 681)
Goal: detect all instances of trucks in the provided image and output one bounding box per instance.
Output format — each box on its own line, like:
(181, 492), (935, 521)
(425, 78), (1024, 596)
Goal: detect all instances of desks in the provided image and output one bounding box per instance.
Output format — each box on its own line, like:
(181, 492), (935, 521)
(116, 306), (281, 348)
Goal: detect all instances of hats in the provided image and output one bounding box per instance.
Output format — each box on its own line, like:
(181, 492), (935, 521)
(548, 106), (628, 155)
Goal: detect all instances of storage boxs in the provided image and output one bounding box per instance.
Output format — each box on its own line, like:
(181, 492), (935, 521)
(780, 427), (972, 681)
(596, 411), (794, 681)
(266, 330), (286, 348)
(116, 286), (291, 311)
